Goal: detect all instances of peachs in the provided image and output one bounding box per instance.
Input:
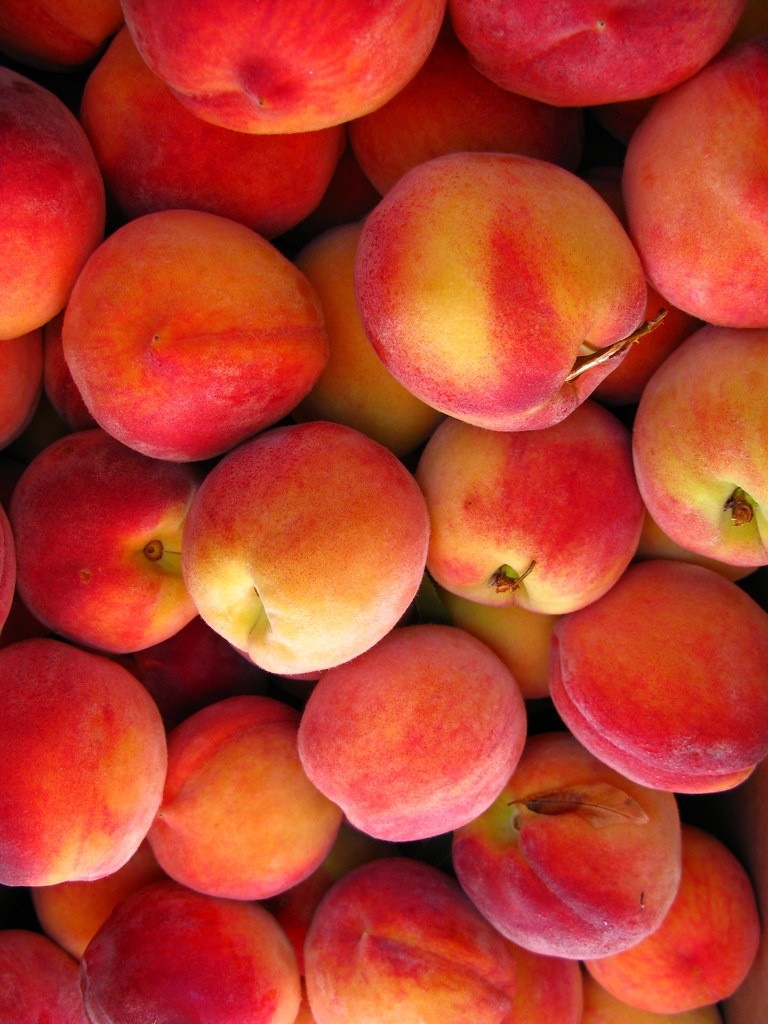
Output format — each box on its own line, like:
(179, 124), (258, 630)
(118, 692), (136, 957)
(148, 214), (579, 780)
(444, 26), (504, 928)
(0, 0), (768, 1024)
